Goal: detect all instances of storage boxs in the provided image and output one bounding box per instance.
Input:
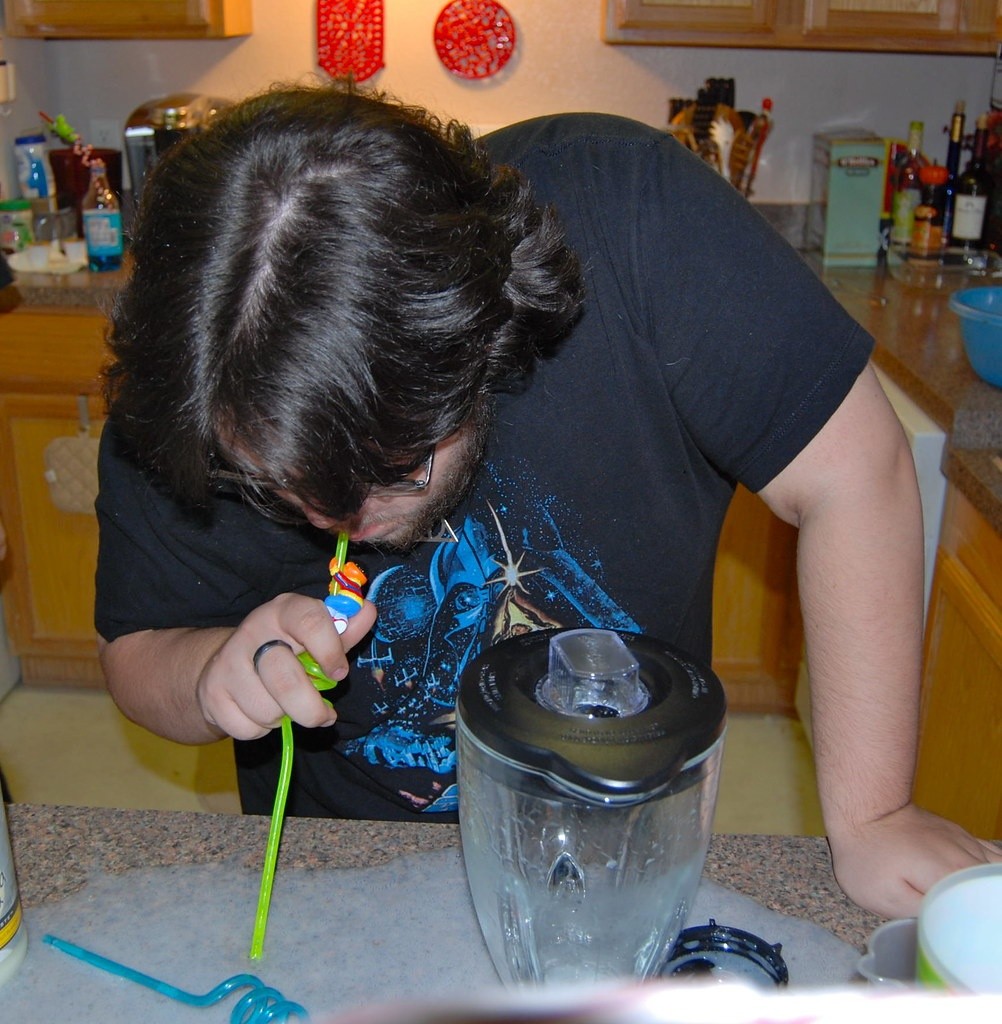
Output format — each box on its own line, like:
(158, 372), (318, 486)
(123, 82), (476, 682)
(804, 130), (890, 264)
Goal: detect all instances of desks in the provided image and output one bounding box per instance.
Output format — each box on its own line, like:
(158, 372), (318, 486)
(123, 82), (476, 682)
(7, 800), (1002, 1024)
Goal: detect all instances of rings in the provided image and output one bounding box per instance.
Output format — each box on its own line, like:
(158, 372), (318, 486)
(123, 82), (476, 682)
(252, 640), (293, 675)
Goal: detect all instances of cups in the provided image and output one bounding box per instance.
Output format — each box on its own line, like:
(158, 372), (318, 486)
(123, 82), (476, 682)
(910, 861), (1002, 996)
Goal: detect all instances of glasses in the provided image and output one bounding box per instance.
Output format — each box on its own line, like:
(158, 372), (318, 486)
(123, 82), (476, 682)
(205, 441), (435, 499)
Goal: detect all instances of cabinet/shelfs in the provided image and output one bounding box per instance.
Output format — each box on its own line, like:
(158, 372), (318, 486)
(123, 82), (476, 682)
(0, 298), (1002, 832)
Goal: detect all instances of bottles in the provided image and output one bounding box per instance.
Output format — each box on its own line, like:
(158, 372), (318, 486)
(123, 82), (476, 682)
(14, 134), (56, 200)
(887, 102), (1002, 268)
(81, 166), (124, 273)
(0, 198), (36, 253)
(0, 787), (27, 989)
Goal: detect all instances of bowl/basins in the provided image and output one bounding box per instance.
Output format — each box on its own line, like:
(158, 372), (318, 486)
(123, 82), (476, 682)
(949, 285), (1001, 391)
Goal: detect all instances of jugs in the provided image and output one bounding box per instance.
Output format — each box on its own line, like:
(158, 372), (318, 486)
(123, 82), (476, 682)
(453, 627), (729, 995)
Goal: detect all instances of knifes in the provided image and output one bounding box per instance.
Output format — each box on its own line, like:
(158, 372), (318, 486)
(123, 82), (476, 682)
(667, 79), (759, 150)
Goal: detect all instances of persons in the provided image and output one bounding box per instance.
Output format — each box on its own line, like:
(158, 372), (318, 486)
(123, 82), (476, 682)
(90, 84), (1002, 924)
